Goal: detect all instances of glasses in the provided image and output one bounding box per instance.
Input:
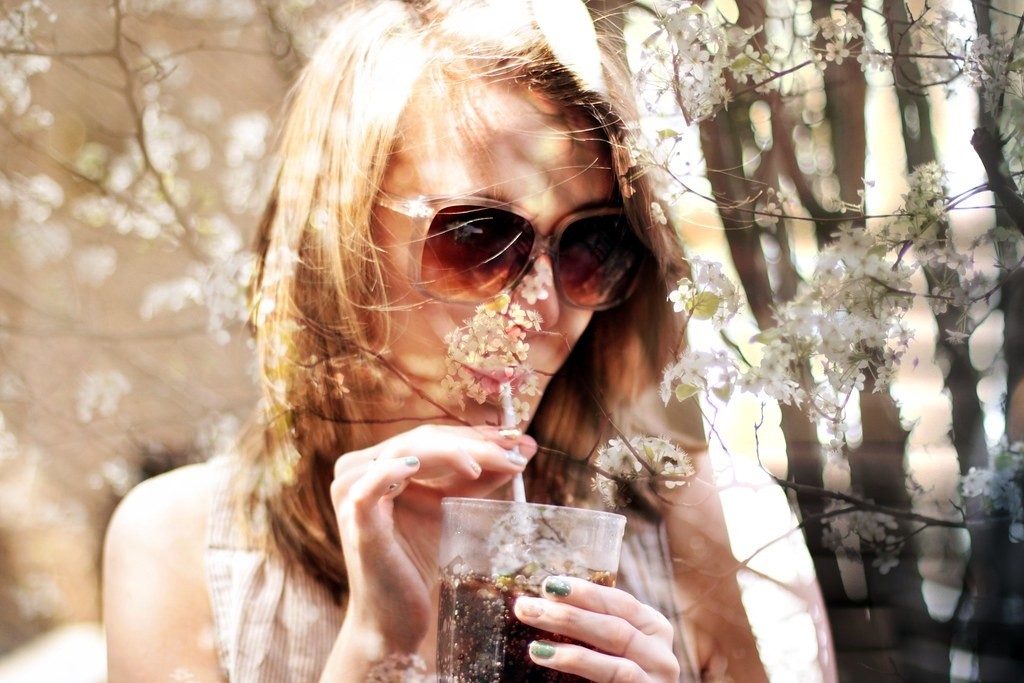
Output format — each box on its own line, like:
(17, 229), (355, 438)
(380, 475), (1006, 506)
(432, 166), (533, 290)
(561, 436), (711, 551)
(373, 193), (653, 309)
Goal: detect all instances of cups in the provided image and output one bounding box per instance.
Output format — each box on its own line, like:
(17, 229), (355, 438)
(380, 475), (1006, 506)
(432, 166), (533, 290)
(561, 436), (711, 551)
(435, 497), (626, 683)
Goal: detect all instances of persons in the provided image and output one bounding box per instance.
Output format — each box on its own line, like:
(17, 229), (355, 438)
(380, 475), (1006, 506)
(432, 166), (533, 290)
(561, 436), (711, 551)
(102, 2), (683, 683)
